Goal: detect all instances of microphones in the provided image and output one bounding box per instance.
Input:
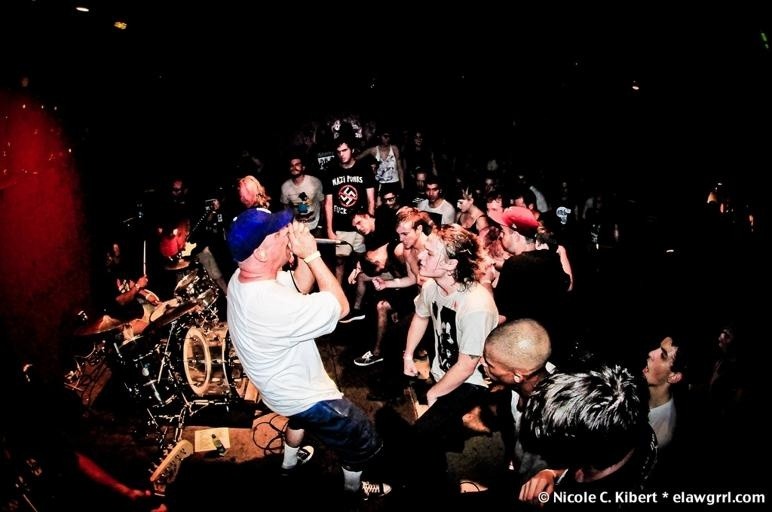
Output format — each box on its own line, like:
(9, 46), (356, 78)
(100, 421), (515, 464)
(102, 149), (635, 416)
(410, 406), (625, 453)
(287, 238), (348, 251)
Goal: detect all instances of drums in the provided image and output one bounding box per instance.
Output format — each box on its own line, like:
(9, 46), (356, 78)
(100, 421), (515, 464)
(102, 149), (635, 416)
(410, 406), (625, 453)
(149, 298), (179, 321)
(174, 270), (218, 303)
(171, 322), (248, 397)
(114, 333), (179, 408)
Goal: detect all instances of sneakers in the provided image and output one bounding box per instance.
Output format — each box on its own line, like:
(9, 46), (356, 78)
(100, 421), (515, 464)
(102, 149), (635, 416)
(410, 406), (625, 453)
(279, 445), (314, 477)
(337, 307), (368, 324)
(353, 349), (384, 368)
(341, 478), (392, 498)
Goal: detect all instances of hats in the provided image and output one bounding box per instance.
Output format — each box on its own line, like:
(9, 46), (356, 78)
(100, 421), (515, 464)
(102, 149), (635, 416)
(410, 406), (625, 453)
(228, 208), (294, 262)
(486, 206), (538, 239)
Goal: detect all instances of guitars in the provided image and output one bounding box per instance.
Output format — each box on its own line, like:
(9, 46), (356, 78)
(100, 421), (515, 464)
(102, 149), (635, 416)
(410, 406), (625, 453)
(177, 203), (214, 257)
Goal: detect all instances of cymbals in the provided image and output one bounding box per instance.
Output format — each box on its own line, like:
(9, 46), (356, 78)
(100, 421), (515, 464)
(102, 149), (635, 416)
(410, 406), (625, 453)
(164, 256), (199, 271)
(71, 314), (126, 336)
(140, 303), (199, 336)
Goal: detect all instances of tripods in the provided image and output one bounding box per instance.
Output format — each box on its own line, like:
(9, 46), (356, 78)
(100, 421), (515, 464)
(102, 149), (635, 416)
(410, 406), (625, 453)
(166, 353), (230, 442)
